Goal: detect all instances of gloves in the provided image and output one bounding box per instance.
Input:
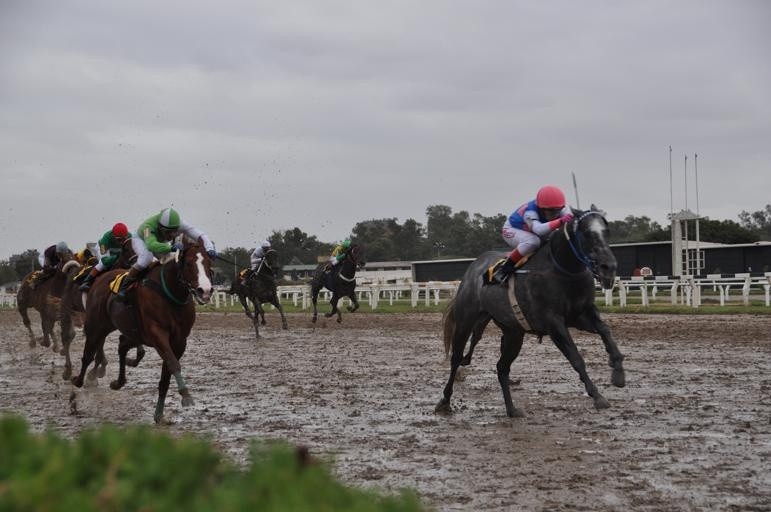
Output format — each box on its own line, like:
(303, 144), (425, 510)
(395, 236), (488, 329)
(171, 244), (184, 252)
(560, 214), (571, 223)
(207, 250), (216, 261)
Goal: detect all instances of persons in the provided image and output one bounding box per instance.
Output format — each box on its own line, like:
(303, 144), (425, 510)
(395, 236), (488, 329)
(325, 239), (352, 274)
(633, 264), (641, 276)
(241, 241), (272, 287)
(28, 241), (72, 291)
(116, 207), (215, 299)
(78, 223), (132, 290)
(75, 248), (95, 267)
(491, 185), (575, 283)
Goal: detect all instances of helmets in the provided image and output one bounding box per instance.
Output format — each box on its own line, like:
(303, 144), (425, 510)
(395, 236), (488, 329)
(56, 242), (67, 253)
(158, 208), (181, 229)
(112, 223), (128, 239)
(537, 185), (566, 209)
(84, 249), (91, 257)
(262, 241), (271, 248)
(342, 240), (350, 248)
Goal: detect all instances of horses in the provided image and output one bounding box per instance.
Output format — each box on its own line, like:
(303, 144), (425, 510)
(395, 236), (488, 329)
(71, 256), (98, 328)
(217, 247), (289, 338)
(17, 249), (76, 356)
(71, 233), (214, 426)
(435, 204), (625, 417)
(61, 237), (145, 386)
(306, 244), (365, 323)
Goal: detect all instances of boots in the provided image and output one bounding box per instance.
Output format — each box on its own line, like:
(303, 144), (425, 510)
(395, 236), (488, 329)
(29, 280), (40, 290)
(242, 269), (254, 287)
(116, 276), (136, 302)
(317, 271), (326, 284)
(77, 273), (95, 291)
(492, 257), (513, 283)
